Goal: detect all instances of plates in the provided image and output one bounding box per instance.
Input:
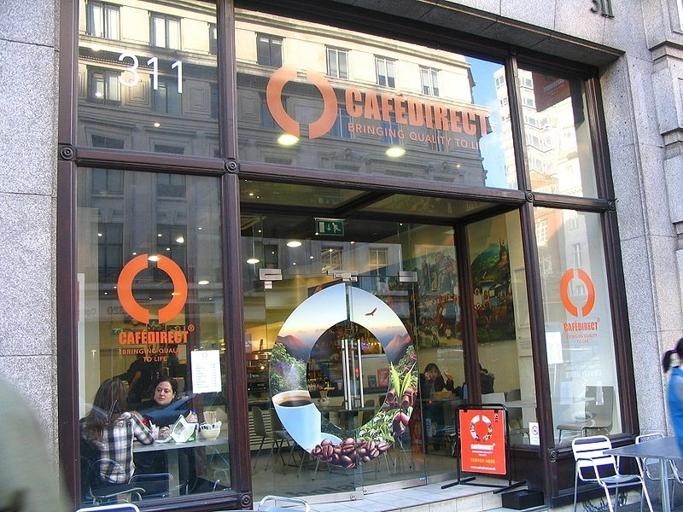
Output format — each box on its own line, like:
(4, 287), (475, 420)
(307, 433), (343, 454)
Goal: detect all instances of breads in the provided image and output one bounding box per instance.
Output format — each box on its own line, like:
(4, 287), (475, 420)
(443, 369), (449, 376)
(441, 387), (449, 393)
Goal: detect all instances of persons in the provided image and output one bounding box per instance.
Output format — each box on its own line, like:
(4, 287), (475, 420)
(126, 347), (151, 402)
(125, 378), (190, 495)
(454, 361), (494, 398)
(80, 376), (156, 497)
(662, 334), (682, 455)
(414, 361), (454, 454)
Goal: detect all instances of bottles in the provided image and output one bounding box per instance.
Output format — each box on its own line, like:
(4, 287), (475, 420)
(149, 424), (170, 440)
(186, 411), (199, 441)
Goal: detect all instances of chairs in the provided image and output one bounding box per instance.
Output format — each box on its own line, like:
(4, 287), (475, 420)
(557, 384), (617, 442)
(571, 431), (683, 512)
(79, 456), (147, 504)
(251, 394), (413, 480)
(479, 389), (524, 432)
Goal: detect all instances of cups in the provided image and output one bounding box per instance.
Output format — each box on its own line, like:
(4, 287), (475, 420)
(272, 390), (321, 450)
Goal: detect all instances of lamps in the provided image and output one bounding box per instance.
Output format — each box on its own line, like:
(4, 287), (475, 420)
(246, 228), (260, 265)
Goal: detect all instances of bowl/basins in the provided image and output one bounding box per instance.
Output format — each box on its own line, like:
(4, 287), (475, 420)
(200, 429), (220, 441)
